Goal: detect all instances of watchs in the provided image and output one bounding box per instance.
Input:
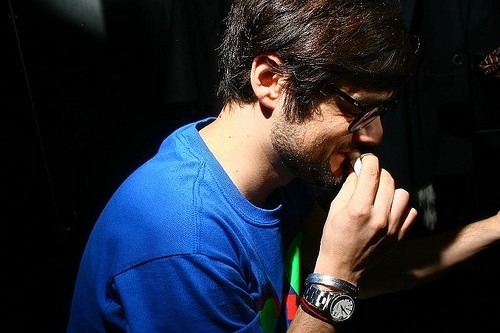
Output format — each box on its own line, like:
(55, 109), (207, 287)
(299, 287), (357, 325)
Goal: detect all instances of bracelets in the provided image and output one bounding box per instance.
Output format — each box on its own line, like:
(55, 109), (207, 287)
(297, 295), (339, 327)
(304, 272), (363, 295)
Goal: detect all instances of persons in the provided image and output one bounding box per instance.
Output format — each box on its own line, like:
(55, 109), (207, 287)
(66, 0), (500, 333)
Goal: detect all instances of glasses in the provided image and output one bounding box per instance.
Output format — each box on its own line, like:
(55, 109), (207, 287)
(325, 82), (398, 132)
